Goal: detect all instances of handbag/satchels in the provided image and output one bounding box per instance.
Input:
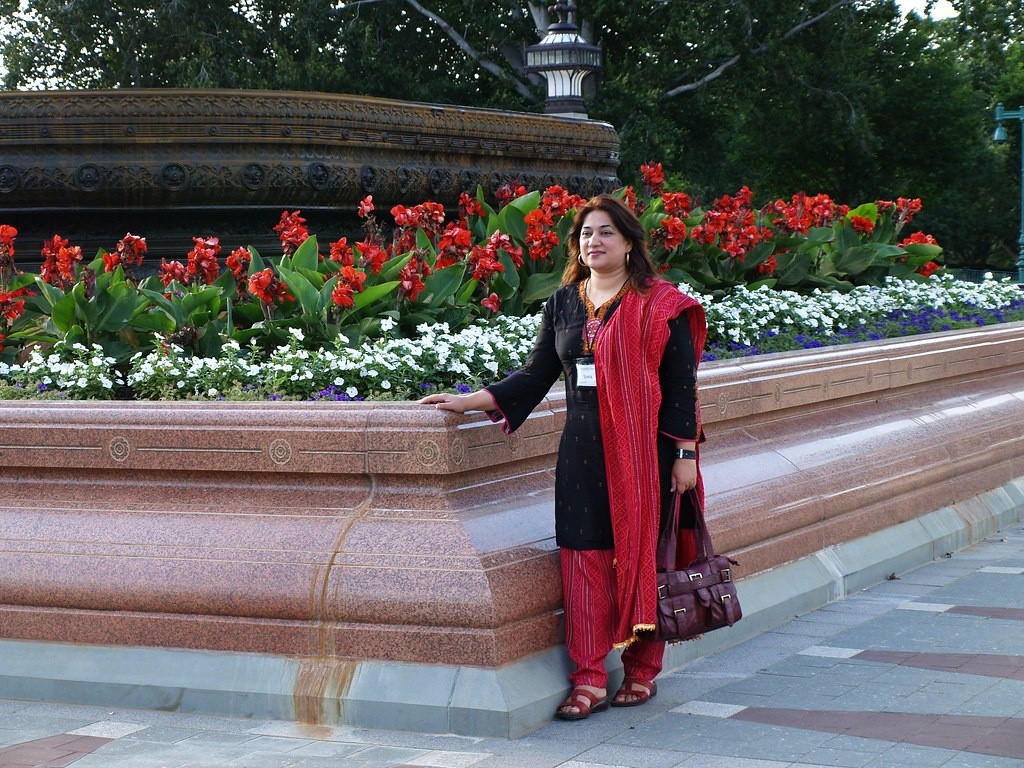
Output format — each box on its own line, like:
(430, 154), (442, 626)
(638, 479), (743, 642)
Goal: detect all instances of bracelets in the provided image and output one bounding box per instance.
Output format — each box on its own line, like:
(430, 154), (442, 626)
(673, 449), (695, 460)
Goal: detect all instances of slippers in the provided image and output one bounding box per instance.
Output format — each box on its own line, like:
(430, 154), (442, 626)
(610, 676), (658, 706)
(556, 689), (610, 720)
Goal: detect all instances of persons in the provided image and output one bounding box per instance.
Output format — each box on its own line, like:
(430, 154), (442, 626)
(419, 192), (706, 723)
